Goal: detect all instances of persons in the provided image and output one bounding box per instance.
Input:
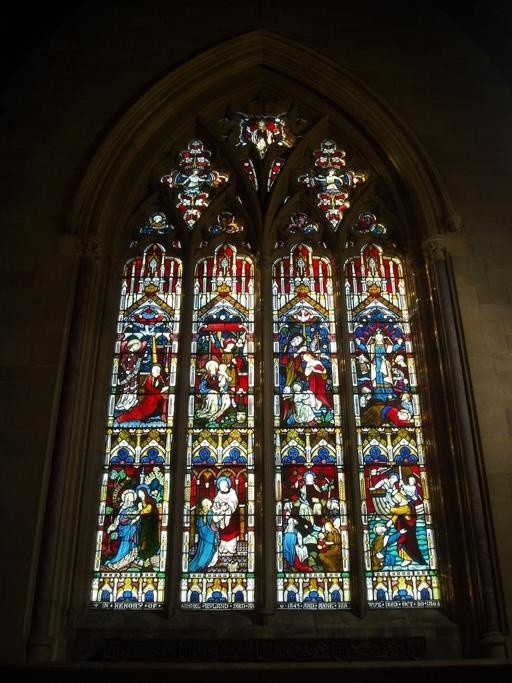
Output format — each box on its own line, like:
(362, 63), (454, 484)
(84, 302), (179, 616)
(315, 171), (343, 189)
(182, 169), (206, 190)
(252, 120), (273, 144)
(151, 215), (162, 226)
